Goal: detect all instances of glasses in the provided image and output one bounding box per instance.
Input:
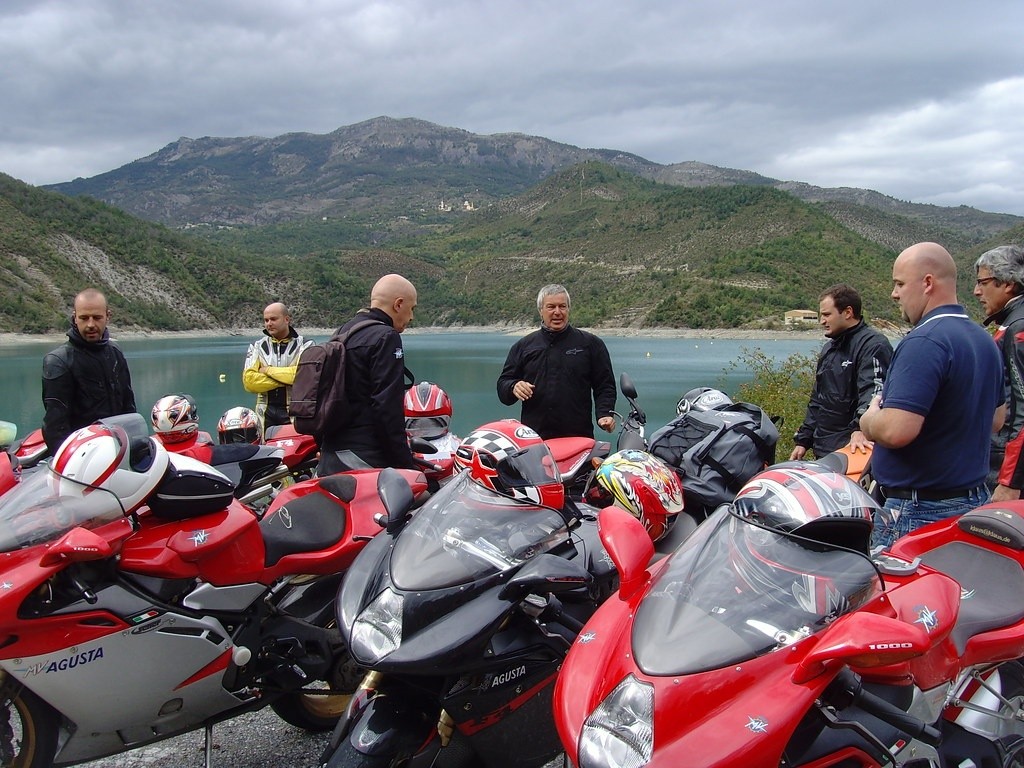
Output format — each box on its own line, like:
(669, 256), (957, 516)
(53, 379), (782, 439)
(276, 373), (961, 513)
(977, 277), (997, 286)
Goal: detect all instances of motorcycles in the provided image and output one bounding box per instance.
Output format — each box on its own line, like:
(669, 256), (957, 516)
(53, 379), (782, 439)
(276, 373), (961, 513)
(318, 418), (709, 768)
(610, 371), (733, 452)
(0, 413), (431, 768)
(0, 392), (320, 517)
(404, 380), (462, 493)
(543, 460), (1024, 768)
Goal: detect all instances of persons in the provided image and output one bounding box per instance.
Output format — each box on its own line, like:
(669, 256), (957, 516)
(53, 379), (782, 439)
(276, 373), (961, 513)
(788, 283), (894, 460)
(242, 302), (316, 445)
(41, 288), (137, 457)
(496, 284), (617, 441)
(974, 245), (1024, 502)
(316, 273), (440, 493)
(860, 241), (1007, 551)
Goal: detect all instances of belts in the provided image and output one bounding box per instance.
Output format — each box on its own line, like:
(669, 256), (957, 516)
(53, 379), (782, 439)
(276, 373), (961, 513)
(881, 487), (978, 501)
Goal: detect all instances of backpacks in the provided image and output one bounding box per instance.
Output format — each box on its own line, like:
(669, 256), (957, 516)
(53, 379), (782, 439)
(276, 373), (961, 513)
(290, 320), (388, 433)
(647, 401), (783, 505)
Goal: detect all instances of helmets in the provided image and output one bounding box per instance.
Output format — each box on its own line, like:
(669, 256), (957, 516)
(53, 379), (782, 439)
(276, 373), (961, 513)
(217, 406), (261, 445)
(404, 381), (453, 440)
(729, 460), (888, 617)
(151, 392), (201, 442)
(453, 419), (564, 510)
(581, 449), (684, 544)
(47, 413), (170, 522)
(677, 386), (733, 416)
